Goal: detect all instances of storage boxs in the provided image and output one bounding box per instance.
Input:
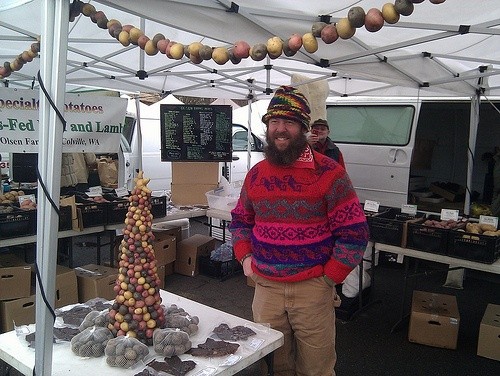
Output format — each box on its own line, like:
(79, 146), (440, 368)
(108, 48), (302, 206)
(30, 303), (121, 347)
(0, 188), (243, 334)
(477, 303), (500, 361)
(408, 290), (460, 349)
(359, 203), (500, 265)
(247, 276), (255, 287)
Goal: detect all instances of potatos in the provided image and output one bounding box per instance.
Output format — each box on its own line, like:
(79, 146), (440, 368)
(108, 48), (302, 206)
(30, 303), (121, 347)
(68, 0), (446, 64)
(71, 305), (198, 368)
(88, 196), (106, 209)
(0, 35), (40, 80)
(419, 219), (500, 243)
(0, 191), (36, 221)
(106, 170), (166, 340)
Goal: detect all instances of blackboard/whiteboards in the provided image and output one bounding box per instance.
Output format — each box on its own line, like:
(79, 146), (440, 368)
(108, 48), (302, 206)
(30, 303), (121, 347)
(160, 105), (232, 162)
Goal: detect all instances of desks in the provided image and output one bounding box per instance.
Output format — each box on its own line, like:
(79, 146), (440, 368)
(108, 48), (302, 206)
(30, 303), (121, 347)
(0, 226), (104, 266)
(206, 209), (374, 324)
(104, 208), (206, 268)
(0, 289), (284, 376)
(374, 242), (500, 334)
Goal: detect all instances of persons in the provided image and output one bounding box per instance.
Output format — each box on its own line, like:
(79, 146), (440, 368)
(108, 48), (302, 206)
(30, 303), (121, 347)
(307, 120), (345, 169)
(72, 153), (96, 192)
(60, 153), (78, 196)
(227, 86), (371, 376)
(87, 158), (108, 187)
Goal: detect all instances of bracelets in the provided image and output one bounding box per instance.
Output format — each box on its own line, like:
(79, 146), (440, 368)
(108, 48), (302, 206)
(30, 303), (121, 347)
(240, 253), (251, 265)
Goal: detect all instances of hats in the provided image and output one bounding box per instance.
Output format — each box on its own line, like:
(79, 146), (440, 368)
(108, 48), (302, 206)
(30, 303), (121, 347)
(310, 119), (329, 130)
(262, 85), (311, 132)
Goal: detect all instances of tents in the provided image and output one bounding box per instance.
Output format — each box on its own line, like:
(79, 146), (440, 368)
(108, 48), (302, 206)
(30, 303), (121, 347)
(0, 0), (500, 376)
